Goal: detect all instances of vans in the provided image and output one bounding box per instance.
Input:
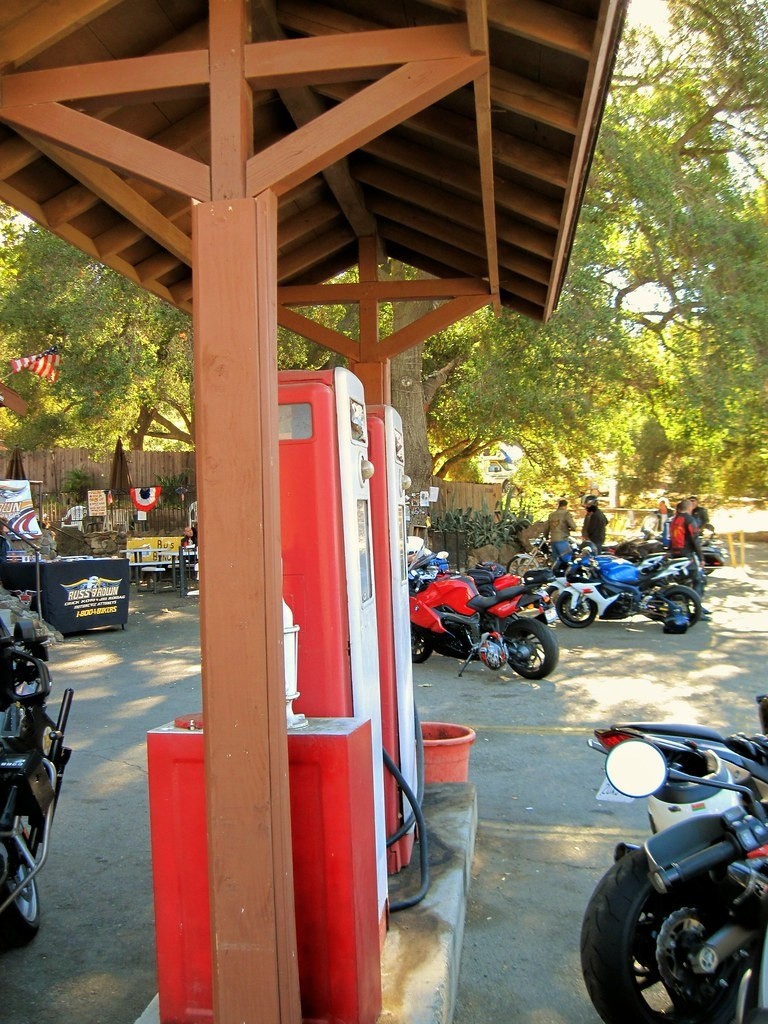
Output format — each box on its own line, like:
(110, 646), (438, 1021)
(473, 443), (519, 492)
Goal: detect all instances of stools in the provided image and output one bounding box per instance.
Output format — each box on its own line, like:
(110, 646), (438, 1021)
(141, 568), (165, 594)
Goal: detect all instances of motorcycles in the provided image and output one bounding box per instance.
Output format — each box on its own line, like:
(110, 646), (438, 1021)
(405, 532), (723, 680)
(581, 692), (768, 1023)
(0, 607), (74, 950)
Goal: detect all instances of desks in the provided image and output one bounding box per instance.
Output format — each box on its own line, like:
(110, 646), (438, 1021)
(60, 555), (89, 558)
(119, 549), (168, 562)
(159, 552), (197, 591)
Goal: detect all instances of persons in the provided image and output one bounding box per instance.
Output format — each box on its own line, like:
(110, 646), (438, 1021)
(654, 495), (712, 621)
(181, 527), (198, 562)
(0, 517), (15, 556)
(581, 494), (608, 555)
(543, 499), (577, 567)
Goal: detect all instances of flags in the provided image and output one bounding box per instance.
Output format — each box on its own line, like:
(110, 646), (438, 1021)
(11, 345), (61, 382)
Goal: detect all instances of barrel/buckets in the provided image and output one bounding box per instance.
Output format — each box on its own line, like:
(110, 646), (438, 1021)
(419, 721), (476, 784)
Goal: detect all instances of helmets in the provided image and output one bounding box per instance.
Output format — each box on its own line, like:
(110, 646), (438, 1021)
(584, 495), (597, 506)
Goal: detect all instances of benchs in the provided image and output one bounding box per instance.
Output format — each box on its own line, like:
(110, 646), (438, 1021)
(130, 559), (196, 590)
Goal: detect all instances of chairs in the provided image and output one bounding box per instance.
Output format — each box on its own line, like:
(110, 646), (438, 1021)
(105, 508), (130, 533)
(59, 505), (88, 533)
(188, 501), (197, 529)
